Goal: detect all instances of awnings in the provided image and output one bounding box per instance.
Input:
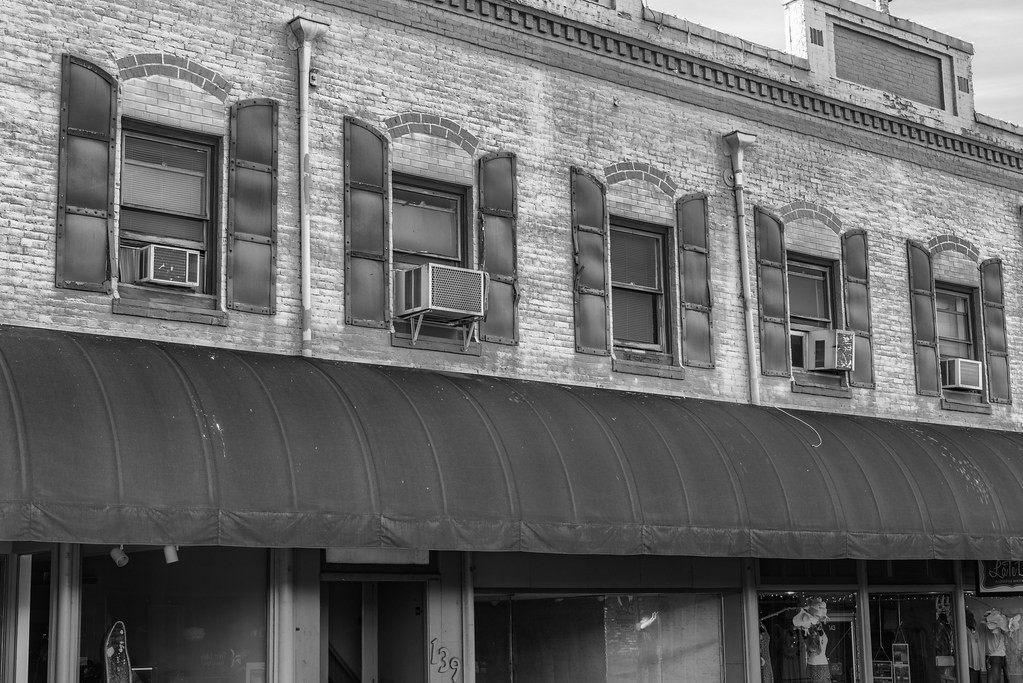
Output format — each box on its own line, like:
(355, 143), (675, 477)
(0, 326), (1022, 560)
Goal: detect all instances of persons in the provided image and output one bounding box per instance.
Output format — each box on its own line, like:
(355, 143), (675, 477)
(965, 610), (1010, 683)
(801, 598), (832, 683)
(759, 623), (774, 683)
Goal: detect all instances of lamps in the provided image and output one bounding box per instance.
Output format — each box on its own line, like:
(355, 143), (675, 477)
(163, 545), (179, 564)
(109, 545), (129, 567)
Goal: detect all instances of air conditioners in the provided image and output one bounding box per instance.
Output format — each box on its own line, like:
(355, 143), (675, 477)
(138, 245), (199, 287)
(806, 330), (856, 371)
(941, 358), (982, 391)
(394, 263), (484, 322)
